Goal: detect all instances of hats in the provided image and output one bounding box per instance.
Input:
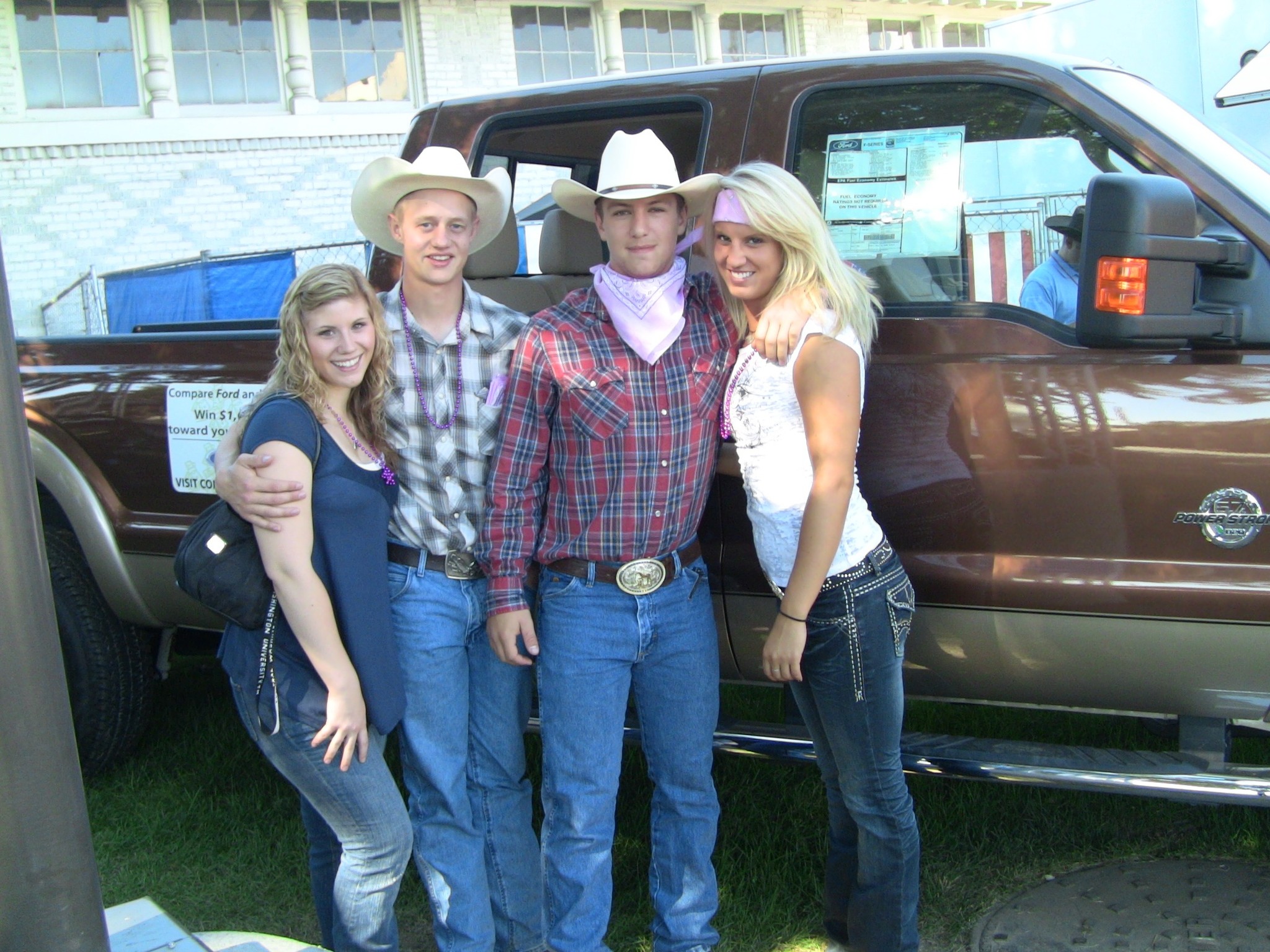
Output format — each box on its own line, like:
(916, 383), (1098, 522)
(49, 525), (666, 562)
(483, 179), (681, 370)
(552, 128), (721, 221)
(1043, 205), (1086, 240)
(352, 145), (511, 256)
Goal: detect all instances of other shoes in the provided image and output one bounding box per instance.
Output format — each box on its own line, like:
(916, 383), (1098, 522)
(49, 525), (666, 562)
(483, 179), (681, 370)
(825, 936), (851, 952)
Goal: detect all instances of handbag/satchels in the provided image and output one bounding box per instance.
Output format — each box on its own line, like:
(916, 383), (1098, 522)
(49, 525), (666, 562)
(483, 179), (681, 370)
(170, 394), (322, 632)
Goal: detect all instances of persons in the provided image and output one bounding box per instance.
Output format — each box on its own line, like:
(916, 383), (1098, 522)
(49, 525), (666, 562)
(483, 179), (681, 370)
(220, 264), (413, 952)
(715, 160), (918, 952)
(213, 146), (548, 952)
(1018, 205), (1088, 328)
(486, 128), (810, 952)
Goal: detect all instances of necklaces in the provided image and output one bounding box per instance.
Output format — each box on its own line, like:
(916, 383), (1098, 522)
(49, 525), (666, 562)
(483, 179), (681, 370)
(720, 348), (759, 440)
(399, 279), (463, 429)
(325, 396), (395, 489)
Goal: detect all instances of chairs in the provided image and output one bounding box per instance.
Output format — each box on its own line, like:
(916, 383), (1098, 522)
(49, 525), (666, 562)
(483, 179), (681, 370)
(461, 206), (551, 320)
(867, 257), (949, 301)
(535, 208), (603, 307)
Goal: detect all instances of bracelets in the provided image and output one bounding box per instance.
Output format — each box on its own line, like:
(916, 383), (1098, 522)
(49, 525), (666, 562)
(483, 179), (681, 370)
(778, 609), (807, 622)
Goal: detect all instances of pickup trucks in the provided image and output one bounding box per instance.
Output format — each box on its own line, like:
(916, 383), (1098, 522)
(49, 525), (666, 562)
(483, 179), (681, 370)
(9, 50), (1270, 802)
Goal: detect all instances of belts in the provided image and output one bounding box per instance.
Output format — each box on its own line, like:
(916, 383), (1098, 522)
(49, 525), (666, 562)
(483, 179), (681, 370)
(387, 540), (486, 580)
(771, 532), (893, 604)
(550, 537), (702, 596)
